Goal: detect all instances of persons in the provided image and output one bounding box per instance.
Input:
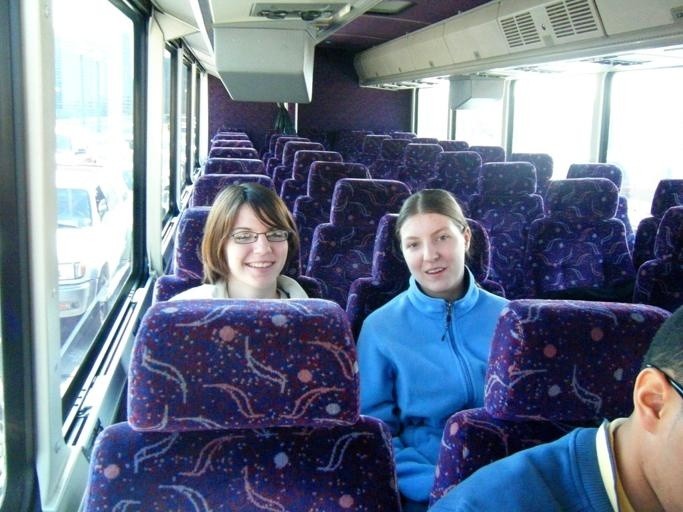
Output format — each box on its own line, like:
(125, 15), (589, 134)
(354, 186), (517, 511)
(423, 301), (681, 510)
(167, 180), (309, 299)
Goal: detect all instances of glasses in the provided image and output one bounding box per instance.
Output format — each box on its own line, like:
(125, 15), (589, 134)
(230, 230), (289, 244)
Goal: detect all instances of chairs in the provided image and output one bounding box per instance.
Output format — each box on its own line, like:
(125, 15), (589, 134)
(86, 128), (683, 511)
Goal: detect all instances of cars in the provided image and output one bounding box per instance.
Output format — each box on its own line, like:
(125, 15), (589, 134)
(52, 110), (195, 335)
